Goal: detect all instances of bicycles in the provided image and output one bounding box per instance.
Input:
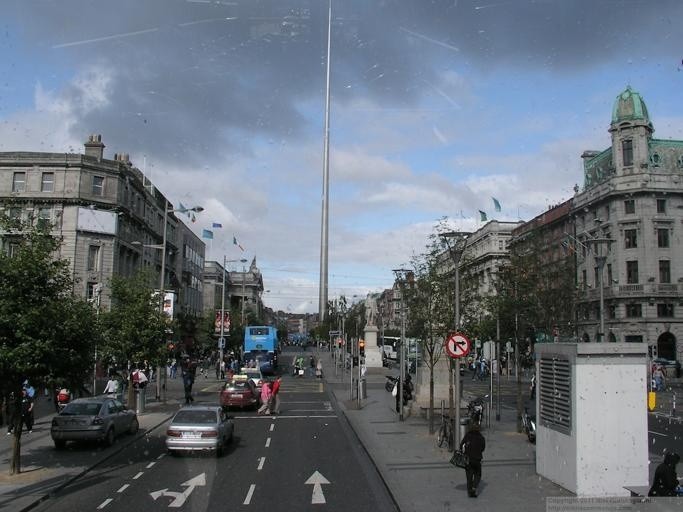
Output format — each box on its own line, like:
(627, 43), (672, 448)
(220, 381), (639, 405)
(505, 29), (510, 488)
(436, 393), (488, 453)
(517, 403), (536, 445)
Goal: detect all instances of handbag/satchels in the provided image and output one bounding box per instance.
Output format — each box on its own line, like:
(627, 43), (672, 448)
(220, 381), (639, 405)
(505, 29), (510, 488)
(392, 385), (398, 397)
(450, 450), (469, 468)
(316, 371), (321, 375)
(299, 370), (304, 375)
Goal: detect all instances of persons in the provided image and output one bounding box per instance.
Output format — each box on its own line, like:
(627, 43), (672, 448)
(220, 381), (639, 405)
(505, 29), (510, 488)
(463, 352), (538, 400)
(14, 378), (35, 426)
(653, 362), (668, 390)
(57, 387), (73, 408)
(459, 422), (486, 498)
(6, 389), (34, 435)
(647, 453), (682, 498)
(127, 337), (325, 418)
(102, 374), (119, 394)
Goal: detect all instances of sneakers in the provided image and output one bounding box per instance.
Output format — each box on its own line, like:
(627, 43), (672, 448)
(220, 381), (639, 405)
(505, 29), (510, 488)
(469, 489), (477, 498)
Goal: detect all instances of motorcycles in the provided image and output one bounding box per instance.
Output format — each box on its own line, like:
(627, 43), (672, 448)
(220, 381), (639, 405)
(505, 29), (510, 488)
(384, 370), (414, 392)
(460, 357), (491, 383)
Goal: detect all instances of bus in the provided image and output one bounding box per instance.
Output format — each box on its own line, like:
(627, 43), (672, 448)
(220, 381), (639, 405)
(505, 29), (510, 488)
(383, 336), (401, 360)
(244, 326), (282, 369)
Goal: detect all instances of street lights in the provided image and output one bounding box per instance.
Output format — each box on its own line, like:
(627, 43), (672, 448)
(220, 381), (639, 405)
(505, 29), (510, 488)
(127, 197), (206, 408)
(393, 268), (413, 423)
(209, 254), (247, 363)
(579, 217), (617, 344)
(84, 282), (101, 395)
(439, 232), (479, 457)
(244, 289), (272, 326)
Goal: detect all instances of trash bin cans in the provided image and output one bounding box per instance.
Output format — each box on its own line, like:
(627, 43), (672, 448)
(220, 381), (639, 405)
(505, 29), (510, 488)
(360, 379), (366, 399)
(134, 389), (146, 414)
(459, 417), (470, 444)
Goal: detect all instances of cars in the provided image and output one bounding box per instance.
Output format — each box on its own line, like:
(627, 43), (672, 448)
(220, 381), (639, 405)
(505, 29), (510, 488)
(165, 406), (236, 457)
(219, 359), (265, 410)
(284, 337), (329, 348)
(49, 398), (140, 447)
